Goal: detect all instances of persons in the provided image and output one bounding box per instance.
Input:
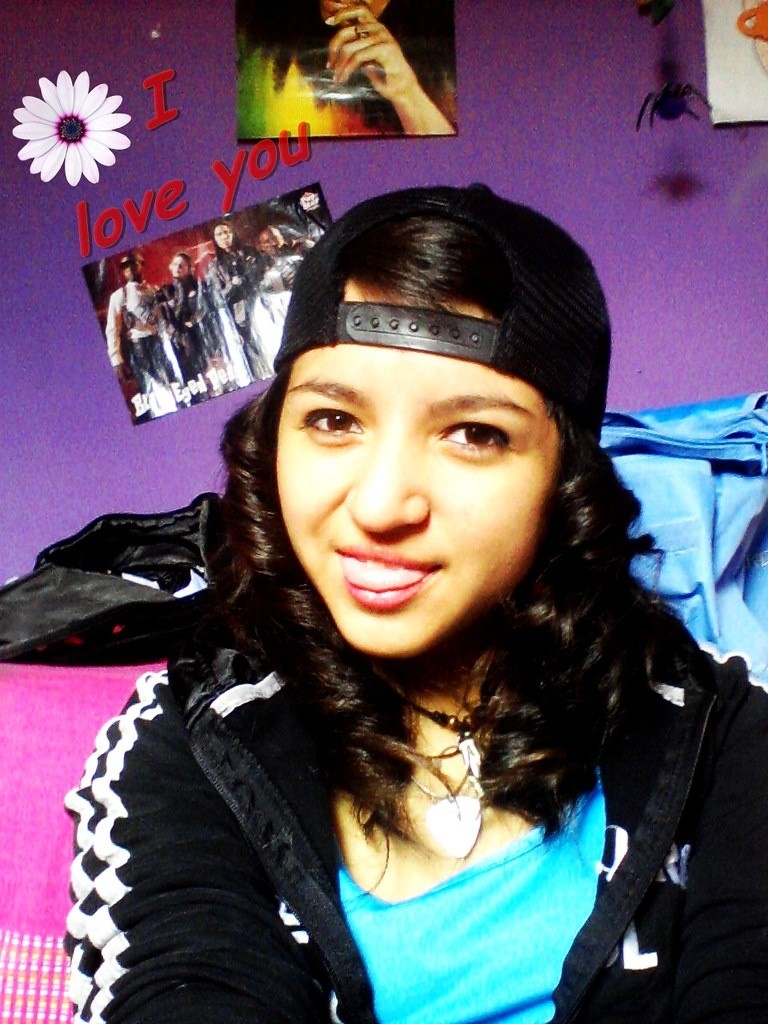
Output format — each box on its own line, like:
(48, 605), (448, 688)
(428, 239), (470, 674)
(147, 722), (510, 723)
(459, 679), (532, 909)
(235, 0), (459, 140)
(62, 183), (768, 1024)
(104, 218), (316, 423)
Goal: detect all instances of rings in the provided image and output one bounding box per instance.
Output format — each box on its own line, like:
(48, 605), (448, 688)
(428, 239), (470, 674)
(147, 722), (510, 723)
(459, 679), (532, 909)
(355, 24), (369, 39)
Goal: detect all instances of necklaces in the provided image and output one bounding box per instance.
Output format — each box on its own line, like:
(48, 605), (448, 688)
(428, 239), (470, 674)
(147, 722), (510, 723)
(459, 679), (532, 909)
(410, 702), (483, 858)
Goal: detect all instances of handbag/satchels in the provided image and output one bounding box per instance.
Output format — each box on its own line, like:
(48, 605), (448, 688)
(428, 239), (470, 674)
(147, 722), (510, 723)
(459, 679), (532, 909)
(591, 390), (768, 685)
(0, 490), (224, 661)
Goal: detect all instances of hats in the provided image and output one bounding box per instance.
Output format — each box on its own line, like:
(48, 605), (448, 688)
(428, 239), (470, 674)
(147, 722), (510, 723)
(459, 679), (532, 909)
(119, 251), (146, 264)
(275, 182), (611, 441)
